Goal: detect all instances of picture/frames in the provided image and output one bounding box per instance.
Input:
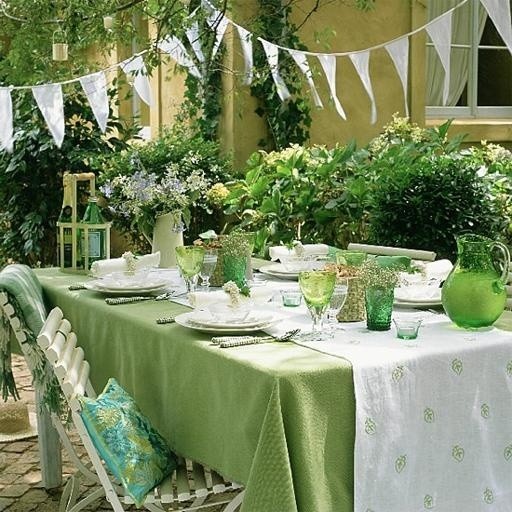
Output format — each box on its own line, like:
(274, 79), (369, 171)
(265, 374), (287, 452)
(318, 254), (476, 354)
(150, 212), (185, 268)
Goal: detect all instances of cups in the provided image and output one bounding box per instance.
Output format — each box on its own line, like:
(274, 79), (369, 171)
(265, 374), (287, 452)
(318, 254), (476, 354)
(392, 316), (421, 340)
(365, 285), (392, 331)
(305, 251), (367, 266)
(281, 289), (302, 306)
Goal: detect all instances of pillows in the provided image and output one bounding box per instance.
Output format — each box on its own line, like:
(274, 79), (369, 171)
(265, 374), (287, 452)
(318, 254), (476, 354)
(77, 378), (179, 509)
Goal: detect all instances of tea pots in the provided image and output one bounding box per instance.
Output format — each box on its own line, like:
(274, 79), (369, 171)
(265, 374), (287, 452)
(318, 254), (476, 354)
(440, 235), (510, 328)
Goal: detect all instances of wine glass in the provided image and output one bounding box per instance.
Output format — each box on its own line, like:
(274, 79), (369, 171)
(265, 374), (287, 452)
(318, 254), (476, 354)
(300, 269), (348, 343)
(175, 247), (218, 294)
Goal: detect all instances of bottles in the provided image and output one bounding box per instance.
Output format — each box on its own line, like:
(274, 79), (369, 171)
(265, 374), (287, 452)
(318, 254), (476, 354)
(59, 180), (103, 268)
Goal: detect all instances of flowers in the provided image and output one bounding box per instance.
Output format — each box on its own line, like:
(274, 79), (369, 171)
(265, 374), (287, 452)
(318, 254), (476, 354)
(88, 123), (239, 233)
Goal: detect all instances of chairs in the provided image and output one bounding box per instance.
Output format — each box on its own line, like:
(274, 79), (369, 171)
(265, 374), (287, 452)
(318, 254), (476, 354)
(36, 307), (245, 512)
(347, 242), (436, 263)
(499, 261), (512, 311)
(0, 289), (122, 512)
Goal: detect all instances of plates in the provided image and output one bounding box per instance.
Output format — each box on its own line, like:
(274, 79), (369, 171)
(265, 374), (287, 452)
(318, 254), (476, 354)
(260, 262), (312, 280)
(83, 277), (169, 295)
(392, 289), (446, 310)
(174, 307), (281, 335)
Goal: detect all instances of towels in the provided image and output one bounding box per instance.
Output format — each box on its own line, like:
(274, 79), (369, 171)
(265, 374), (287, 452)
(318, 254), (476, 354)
(90, 251), (160, 277)
(269, 242), (329, 260)
(188, 288), (273, 307)
(394, 258), (453, 286)
